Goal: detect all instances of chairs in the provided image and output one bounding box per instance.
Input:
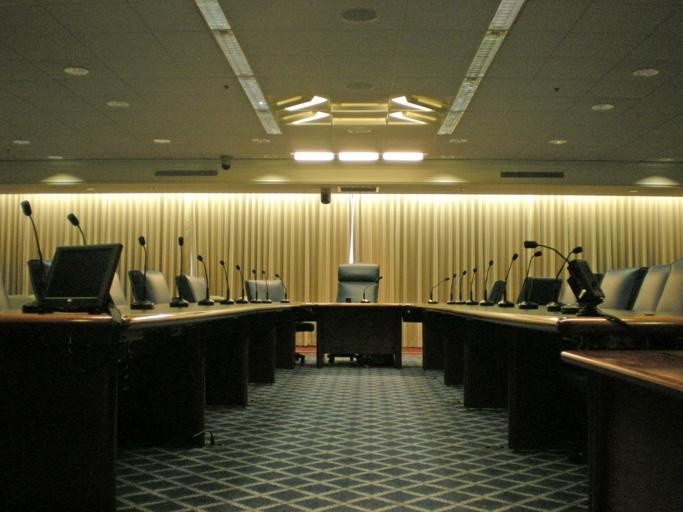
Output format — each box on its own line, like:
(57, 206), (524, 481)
(28, 256), (207, 306)
(244, 279), (284, 304)
(335, 264), (379, 303)
(488, 260), (683, 317)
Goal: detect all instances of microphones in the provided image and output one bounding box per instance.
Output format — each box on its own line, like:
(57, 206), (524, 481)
(480, 260), (495, 306)
(67, 212), (115, 314)
(361, 276), (383, 303)
(547, 246), (582, 312)
(428, 268), (478, 304)
(524, 240), (570, 264)
(196, 254), (290, 304)
(169, 237), (189, 307)
(130, 236), (155, 309)
(518, 250), (542, 309)
(21, 199), (53, 313)
(498, 253), (518, 307)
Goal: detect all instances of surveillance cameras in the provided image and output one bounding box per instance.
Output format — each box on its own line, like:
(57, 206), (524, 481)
(220, 156), (231, 170)
(320, 191), (331, 205)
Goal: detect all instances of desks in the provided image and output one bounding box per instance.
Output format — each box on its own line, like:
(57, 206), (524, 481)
(0, 301), (316, 510)
(560, 348), (681, 510)
(308, 300), (409, 370)
(422, 304), (682, 465)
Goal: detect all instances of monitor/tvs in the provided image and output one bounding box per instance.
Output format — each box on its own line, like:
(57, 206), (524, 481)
(42, 243), (124, 312)
(567, 259), (613, 317)
